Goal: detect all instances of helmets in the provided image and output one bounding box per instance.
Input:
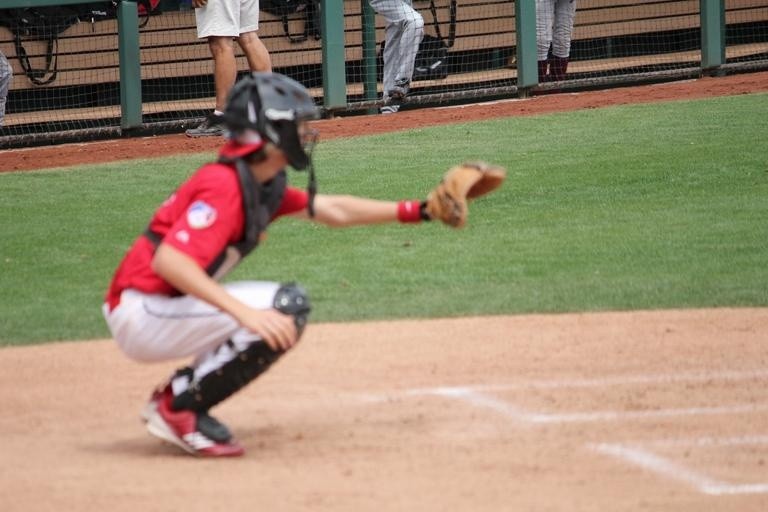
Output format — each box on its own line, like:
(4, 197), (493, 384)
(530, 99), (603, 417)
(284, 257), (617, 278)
(225, 74), (318, 145)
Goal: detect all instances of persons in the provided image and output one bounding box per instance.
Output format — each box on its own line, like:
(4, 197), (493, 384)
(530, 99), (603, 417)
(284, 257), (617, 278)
(536, 0), (576, 82)
(0, 50), (14, 123)
(367, 0), (424, 114)
(187, 0), (270, 136)
(102, 72), (506, 457)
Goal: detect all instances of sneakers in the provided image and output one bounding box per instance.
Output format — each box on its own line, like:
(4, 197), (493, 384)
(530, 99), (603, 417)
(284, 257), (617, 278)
(186, 113), (225, 138)
(142, 383), (246, 456)
(388, 80), (409, 99)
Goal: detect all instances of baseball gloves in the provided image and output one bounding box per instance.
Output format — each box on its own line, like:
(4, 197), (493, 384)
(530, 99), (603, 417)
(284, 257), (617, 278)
(426, 159), (506, 228)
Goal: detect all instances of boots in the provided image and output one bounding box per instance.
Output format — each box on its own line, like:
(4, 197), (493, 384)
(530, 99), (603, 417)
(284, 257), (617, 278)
(532, 55), (569, 95)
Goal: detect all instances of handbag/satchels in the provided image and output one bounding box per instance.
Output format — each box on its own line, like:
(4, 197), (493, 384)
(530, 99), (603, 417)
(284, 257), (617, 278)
(259, 0), (320, 42)
(0, 0), (163, 85)
(378, 34), (447, 81)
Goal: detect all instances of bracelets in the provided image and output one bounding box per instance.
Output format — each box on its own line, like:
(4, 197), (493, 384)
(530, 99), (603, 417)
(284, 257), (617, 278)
(398, 200), (419, 223)
